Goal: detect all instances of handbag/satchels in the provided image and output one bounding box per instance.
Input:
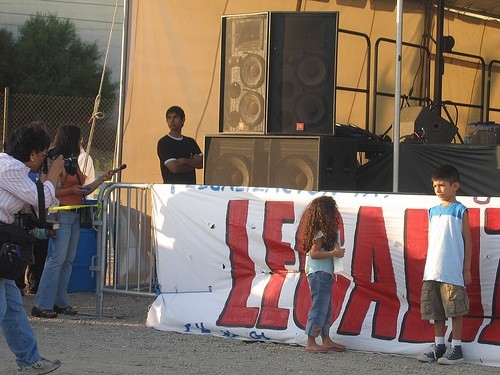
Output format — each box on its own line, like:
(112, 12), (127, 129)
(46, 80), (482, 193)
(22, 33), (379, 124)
(79, 202), (93, 229)
(0, 224), (35, 279)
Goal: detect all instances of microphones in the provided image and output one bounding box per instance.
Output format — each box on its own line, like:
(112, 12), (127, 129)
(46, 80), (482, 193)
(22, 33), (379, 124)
(110, 164), (128, 175)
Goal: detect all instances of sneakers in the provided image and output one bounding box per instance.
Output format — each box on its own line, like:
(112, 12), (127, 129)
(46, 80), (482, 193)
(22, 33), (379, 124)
(417, 343), (447, 362)
(17, 359), (62, 375)
(437, 345), (464, 364)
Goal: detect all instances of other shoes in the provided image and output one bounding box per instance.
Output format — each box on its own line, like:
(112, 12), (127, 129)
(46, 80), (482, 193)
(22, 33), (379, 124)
(305, 346), (328, 353)
(323, 344), (346, 352)
(54, 304), (77, 315)
(31, 306), (57, 318)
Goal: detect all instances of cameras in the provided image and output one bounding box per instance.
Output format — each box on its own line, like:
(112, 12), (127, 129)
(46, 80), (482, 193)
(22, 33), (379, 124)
(43, 148), (78, 176)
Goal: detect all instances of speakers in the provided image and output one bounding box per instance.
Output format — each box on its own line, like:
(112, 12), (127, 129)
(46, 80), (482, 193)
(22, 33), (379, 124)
(398, 106), (458, 144)
(203, 11), (391, 196)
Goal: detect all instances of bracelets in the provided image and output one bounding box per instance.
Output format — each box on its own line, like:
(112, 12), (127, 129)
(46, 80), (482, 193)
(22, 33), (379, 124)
(98, 176), (103, 184)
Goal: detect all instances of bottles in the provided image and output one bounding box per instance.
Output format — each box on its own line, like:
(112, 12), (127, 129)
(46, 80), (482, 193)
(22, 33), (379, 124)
(464, 121), (496, 146)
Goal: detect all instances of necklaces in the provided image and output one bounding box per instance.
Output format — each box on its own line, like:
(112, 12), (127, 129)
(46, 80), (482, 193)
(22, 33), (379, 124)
(439, 202), (455, 217)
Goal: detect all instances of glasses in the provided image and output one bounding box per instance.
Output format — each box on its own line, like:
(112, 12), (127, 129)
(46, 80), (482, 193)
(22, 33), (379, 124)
(79, 137), (83, 143)
(36, 151), (49, 157)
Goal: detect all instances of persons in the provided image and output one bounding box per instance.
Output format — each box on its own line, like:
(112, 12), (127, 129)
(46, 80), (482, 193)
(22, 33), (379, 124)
(418, 163), (472, 366)
(0, 127), (63, 375)
(78, 137), (95, 200)
(303, 196), (350, 353)
(31, 124), (115, 317)
(157, 105), (203, 184)
(15, 170), (61, 294)
